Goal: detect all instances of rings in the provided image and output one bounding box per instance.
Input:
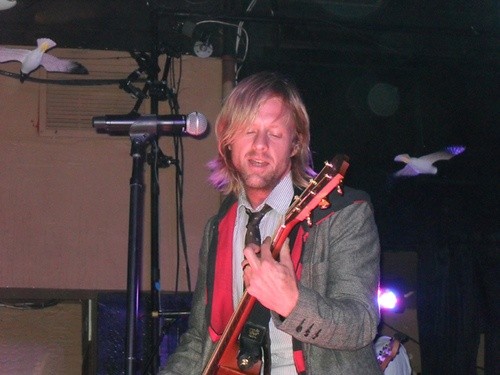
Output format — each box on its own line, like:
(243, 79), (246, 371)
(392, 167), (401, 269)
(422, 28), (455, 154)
(241, 264), (251, 270)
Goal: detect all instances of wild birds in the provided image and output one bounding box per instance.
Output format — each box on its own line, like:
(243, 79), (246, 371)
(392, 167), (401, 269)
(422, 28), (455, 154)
(0, 38), (89, 84)
(394, 146), (466, 177)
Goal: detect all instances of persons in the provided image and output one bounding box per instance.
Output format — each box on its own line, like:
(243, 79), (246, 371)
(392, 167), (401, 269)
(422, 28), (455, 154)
(161, 71), (386, 375)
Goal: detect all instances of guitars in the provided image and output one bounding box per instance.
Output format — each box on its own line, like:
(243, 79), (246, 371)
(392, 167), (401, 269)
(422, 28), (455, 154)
(199, 153), (351, 374)
(376, 330), (411, 374)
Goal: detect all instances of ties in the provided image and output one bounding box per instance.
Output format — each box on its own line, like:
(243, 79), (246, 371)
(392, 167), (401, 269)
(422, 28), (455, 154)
(246, 203), (273, 375)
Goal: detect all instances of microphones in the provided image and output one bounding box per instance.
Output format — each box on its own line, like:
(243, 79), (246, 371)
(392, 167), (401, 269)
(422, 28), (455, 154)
(92, 111), (208, 137)
